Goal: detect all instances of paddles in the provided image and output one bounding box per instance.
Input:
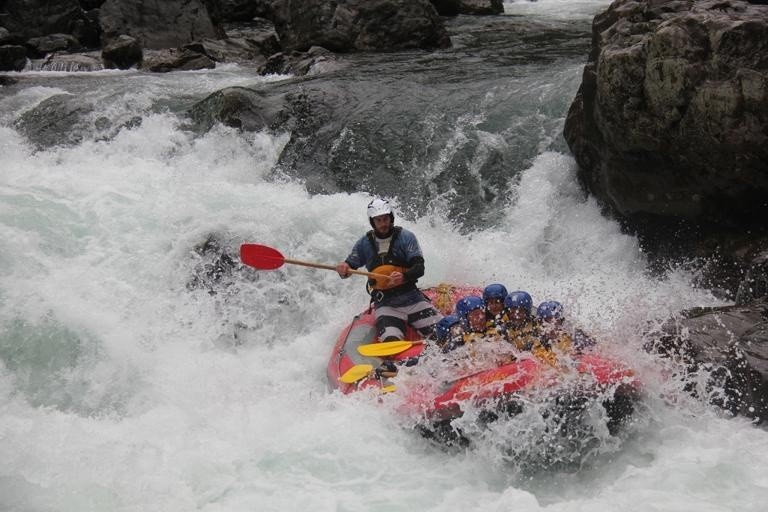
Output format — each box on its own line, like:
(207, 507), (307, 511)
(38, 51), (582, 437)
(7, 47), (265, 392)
(357, 339), (424, 357)
(340, 364), (398, 384)
(240, 243), (391, 281)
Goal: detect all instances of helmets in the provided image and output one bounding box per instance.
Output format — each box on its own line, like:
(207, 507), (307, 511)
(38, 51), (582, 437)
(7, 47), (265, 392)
(435, 315), (465, 342)
(483, 284), (508, 307)
(533, 301), (564, 326)
(454, 295), (487, 327)
(366, 198), (395, 228)
(503, 291), (533, 317)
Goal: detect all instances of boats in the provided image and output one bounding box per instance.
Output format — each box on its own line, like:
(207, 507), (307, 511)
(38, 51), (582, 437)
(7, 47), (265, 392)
(327, 284), (645, 470)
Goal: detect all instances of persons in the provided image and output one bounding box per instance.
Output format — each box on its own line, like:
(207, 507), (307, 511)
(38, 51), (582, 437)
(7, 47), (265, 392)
(336, 199), (444, 360)
(374, 284), (598, 395)
